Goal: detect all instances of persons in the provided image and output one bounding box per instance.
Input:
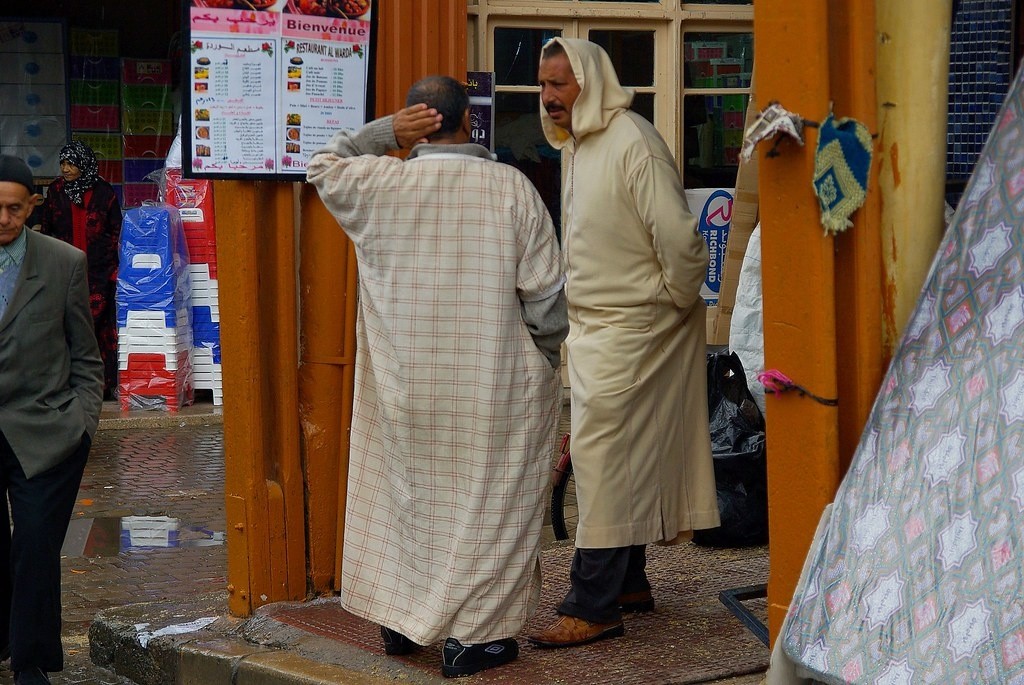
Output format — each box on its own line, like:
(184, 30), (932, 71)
(0, 153), (105, 685)
(39, 141), (118, 404)
(304, 77), (570, 674)
(528, 35), (720, 646)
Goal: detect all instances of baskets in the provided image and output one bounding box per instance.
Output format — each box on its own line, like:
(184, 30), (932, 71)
(70, 26), (172, 206)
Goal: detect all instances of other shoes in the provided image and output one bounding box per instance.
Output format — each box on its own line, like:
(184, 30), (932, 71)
(556, 589), (654, 615)
(381, 625), (423, 656)
(13, 667), (51, 684)
(441, 638), (518, 678)
(528, 615), (625, 648)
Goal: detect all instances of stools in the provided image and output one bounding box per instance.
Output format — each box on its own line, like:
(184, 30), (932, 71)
(116, 168), (223, 413)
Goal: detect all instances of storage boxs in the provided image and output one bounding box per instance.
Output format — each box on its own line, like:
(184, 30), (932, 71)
(686, 40), (752, 165)
(0, 13), (175, 207)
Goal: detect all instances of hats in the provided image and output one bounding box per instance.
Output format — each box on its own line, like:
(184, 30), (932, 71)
(0, 154), (33, 194)
